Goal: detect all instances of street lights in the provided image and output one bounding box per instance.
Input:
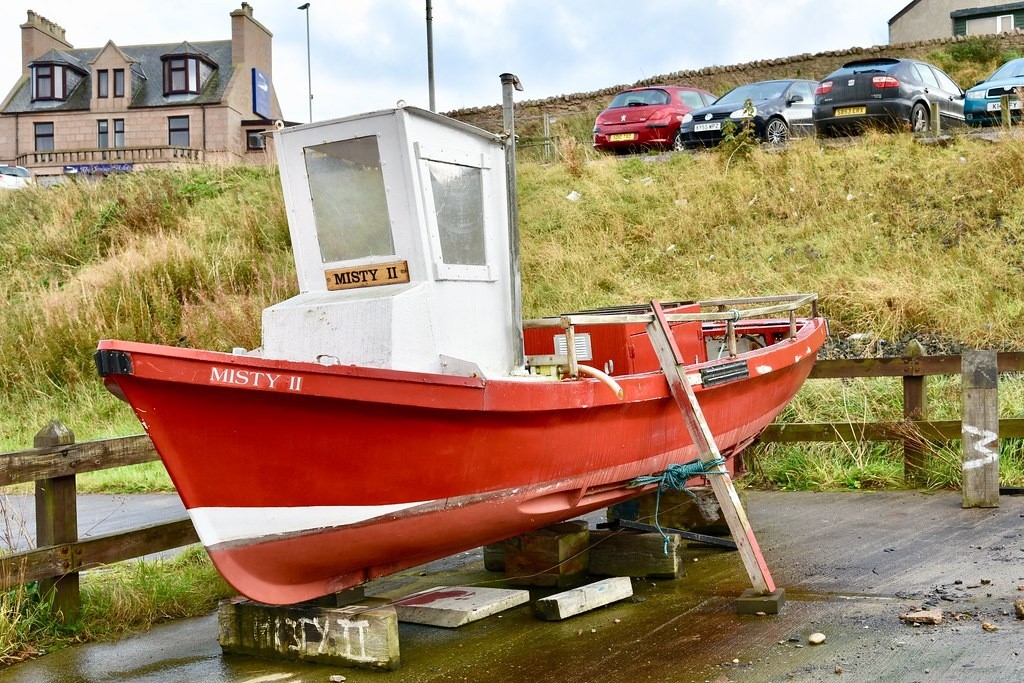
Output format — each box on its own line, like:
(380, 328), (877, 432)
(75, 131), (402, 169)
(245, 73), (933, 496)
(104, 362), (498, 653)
(297, 2), (313, 124)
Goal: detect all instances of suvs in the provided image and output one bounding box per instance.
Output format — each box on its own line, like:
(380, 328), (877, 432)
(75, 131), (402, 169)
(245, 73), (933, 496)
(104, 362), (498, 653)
(812, 55), (966, 139)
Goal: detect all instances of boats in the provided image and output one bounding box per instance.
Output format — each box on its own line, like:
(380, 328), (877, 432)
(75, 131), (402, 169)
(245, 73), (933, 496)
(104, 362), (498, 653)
(94, 0), (828, 606)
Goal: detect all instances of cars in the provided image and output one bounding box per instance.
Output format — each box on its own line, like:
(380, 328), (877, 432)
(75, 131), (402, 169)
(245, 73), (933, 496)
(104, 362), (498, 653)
(679, 79), (821, 150)
(0, 164), (34, 189)
(962, 57), (1024, 129)
(591, 84), (721, 155)
